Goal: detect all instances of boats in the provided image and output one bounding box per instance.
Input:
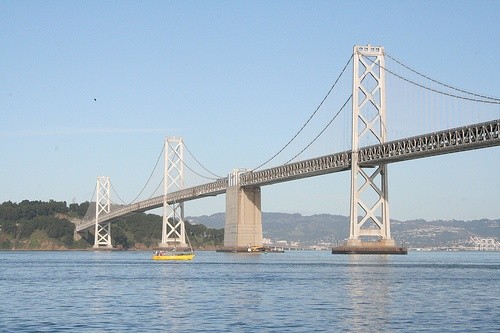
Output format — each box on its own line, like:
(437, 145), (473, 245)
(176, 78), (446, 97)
(153, 252), (195, 260)
